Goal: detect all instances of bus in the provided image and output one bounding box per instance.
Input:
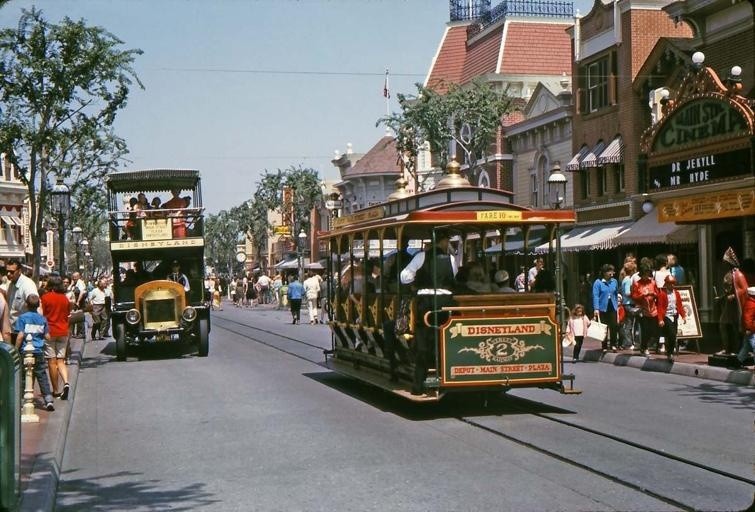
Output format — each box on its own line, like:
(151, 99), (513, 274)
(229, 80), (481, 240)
(104, 168), (213, 362)
(317, 182), (581, 406)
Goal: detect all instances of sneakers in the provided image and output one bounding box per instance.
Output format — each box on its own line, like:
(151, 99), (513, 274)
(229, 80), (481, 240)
(315, 318), (319, 324)
(573, 358), (579, 364)
(98, 338), (107, 340)
(76, 335), (86, 339)
(46, 400), (55, 411)
(320, 320), (323, 324)
(103, 335), (112, 337)
(91, 335), (96, 341)
(297, 319), (301, 325)
(603, 348), (608, 353)
(309, 320), (314, 325)
(640, 349), (651, 357)
(612, 346), (617, 353)
(60, 383), (70, 400)
(292, 318), (297, 325)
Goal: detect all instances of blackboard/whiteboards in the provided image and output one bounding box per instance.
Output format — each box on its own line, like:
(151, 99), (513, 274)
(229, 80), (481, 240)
(659, 285), (703, 339)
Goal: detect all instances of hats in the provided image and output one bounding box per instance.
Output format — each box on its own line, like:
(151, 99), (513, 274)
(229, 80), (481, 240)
(665, 275), (677, 283)
(495, 270), (509, 283)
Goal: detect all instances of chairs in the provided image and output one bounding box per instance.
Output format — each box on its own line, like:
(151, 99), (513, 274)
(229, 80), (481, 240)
(326, 292), (417, 364)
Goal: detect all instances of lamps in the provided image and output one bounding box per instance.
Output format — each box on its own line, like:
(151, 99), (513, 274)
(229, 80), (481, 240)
(690, 50), (706, 72)
(640, 199), (655, 214)
(658, 88), (671, 106)
(729, 64), (744, 85)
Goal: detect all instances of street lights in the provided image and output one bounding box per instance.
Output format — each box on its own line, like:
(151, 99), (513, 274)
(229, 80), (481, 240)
(51, 179), (94, 306)
(548, 169), (570, 336)
(298, 228), (306, 281)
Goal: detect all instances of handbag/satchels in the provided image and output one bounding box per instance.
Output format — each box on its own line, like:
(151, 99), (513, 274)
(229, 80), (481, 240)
(67, 309), (86, 324)
(585, 311), (608, 342)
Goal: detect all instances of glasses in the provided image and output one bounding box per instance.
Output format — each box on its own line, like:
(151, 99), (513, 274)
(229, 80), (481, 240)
(6, 268), (19, 274)
